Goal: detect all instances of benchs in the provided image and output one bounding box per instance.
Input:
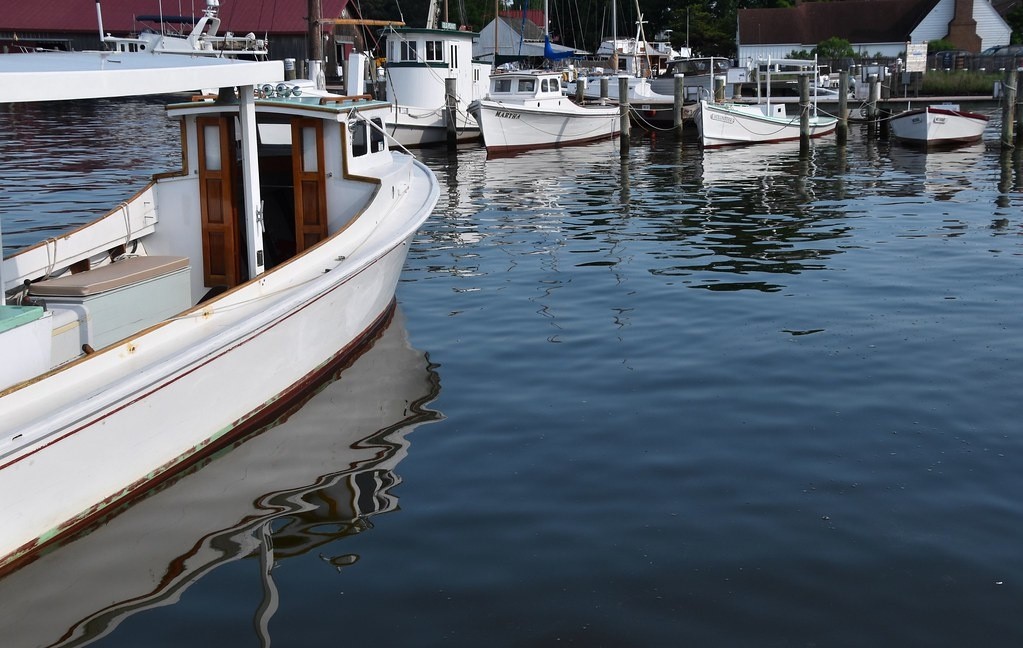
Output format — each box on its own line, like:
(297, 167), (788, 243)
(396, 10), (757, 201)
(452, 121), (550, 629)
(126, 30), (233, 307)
(28, 257), (191, 355)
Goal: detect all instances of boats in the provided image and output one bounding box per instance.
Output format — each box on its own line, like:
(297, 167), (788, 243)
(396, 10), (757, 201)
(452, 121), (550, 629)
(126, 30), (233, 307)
(466, 93), (632, 153)
(4, 296), (445, 645)
(0, 0), (286, 99)
(889, 104), (991, 150)
(2, 81), (440, 591)
(695, 97), (839, 150)
(228, 0), (750, 153)
